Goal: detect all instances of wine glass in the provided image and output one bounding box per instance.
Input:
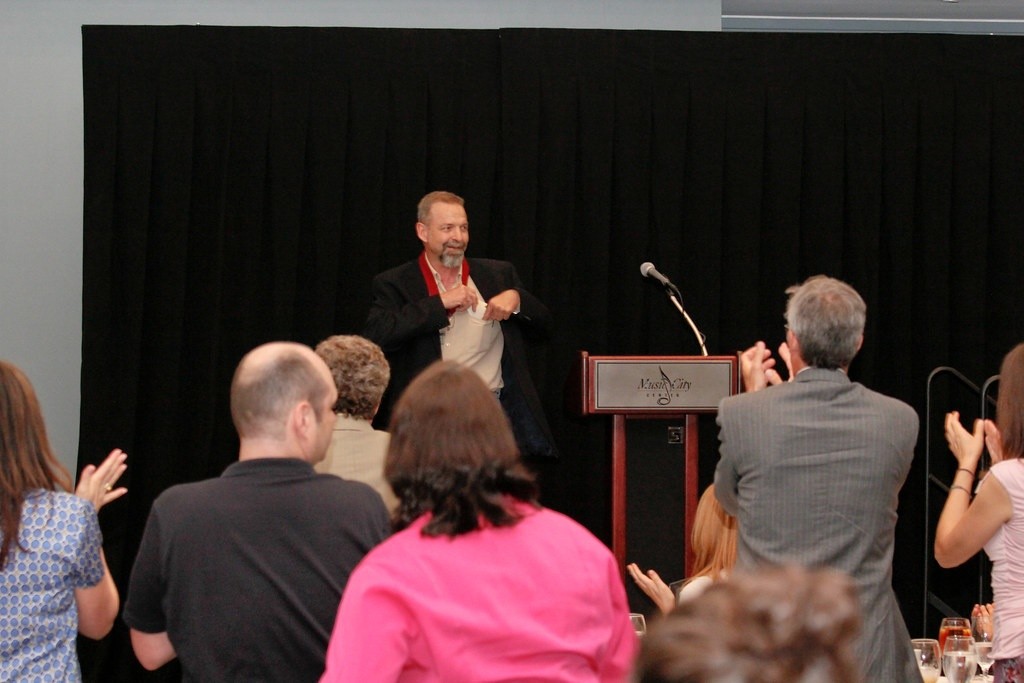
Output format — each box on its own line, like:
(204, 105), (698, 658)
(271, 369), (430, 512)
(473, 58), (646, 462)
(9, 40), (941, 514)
(971, 617), (995, 681)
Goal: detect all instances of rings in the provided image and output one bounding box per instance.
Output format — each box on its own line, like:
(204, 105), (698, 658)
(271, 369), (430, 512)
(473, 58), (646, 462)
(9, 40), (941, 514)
(104, 483), (113, 491)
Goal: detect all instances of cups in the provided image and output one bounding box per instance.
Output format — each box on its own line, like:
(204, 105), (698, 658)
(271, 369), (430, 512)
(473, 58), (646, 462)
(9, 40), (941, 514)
(939, 618), (971, 666)
(942, 636), (976, 683)
(629, 613), (647, 639)
(911, 638), (941, 683)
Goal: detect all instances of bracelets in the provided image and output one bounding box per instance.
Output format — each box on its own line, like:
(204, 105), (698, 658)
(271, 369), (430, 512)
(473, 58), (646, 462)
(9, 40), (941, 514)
(950, 484), (972, 498)
(957, 468), (975, 478)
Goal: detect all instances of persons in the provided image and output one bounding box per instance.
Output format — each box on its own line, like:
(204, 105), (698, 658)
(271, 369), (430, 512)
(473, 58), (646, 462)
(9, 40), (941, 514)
(935, 344), (1024, 682)
(625, 484), (737, 614)
(320, 359), (640, 683)
(360, 192), (556, 507)
(715, 274), (926, 683)
(640, 563), (861, 683)
(315, 334), (402, 535)
(0, 360), (128, 683)
(130, 342), (391, 683)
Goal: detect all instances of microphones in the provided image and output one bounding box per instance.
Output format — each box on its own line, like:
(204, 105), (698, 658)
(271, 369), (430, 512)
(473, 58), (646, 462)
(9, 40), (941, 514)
(640, 262), (678, 292)
